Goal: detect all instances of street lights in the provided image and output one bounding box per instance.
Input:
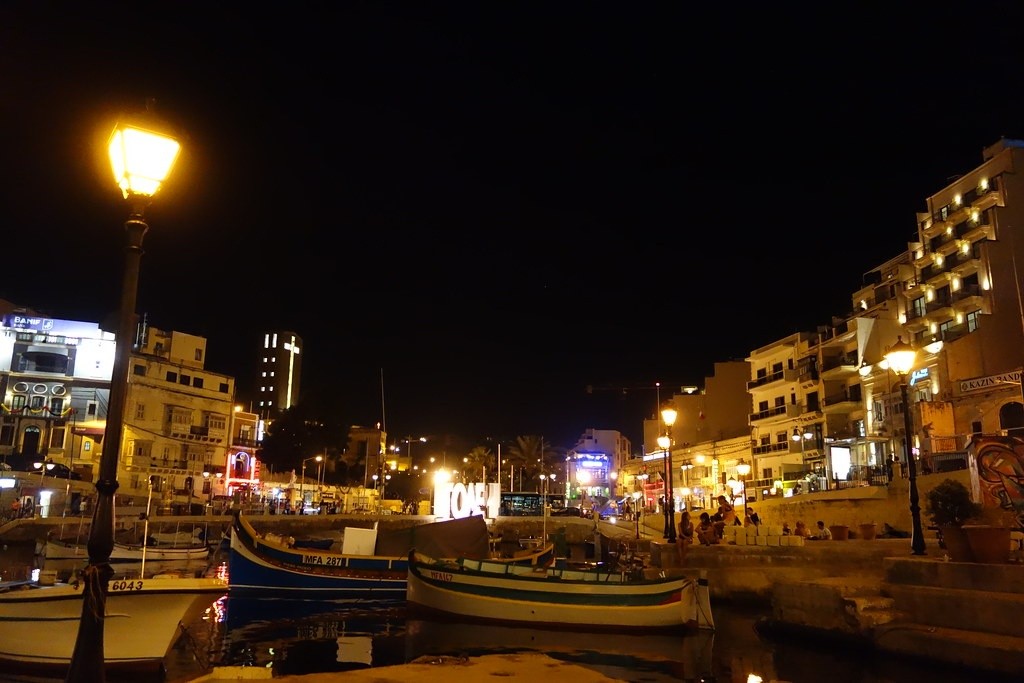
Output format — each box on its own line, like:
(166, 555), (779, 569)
(735, 457), (751, 517)
(299, 455), (322, 509)
(408, 435), (426, 458)
(882, 334), (927, 556)
(66, 120), (186, 683)
(681, 459), (692, 501)
(371, 475), (378, 490)
(790, 427), (813, 480)
(660, 408), (678, 544)
(656, 429), (670, 539)
(633, 491), (641, 539)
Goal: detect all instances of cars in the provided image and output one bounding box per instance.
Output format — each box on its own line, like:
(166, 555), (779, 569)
(155, 495), (319, 517)
(501, 506), (604, 520)
(26, 461), (82, 481)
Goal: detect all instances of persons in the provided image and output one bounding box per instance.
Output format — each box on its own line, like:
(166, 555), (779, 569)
(922, 449), (931, 475)
(11, 498), (33, 518)
(71, 494), (88, 516)
(623, 496), (636, 522)
(262, 496), (345, 514)
(608, 535), (629, 572)
(658, 496), (665, 515)
(807, 470), (818, 492)
(783, 520), (832, 541)
(695, 495), (741, 546)
(745, 507), (760, 527)
(886, 454), (903, 481)
(678, 511), (694, 566)
(792, 479), (802, 496)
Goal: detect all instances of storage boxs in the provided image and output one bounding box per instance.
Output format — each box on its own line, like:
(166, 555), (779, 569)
(724, 525), (806, 547)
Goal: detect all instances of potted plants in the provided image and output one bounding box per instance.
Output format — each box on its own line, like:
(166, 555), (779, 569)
(923, 478), (982, 555)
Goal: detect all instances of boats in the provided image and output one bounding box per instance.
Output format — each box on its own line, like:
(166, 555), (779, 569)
(0, 485), (229, 673)
(31, 536), (210, 560)
(406, 549), (698, 636)
(438, 554), (715, 633)
(226, 506), (554, 598)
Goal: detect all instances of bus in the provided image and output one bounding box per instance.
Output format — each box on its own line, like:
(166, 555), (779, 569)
(500, 492), (567, 513)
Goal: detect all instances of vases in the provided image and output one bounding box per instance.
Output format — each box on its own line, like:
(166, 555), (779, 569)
(829, 525), (849, 540)
(858, 524), (877, 540)
(960, 523), (1014, 565)
